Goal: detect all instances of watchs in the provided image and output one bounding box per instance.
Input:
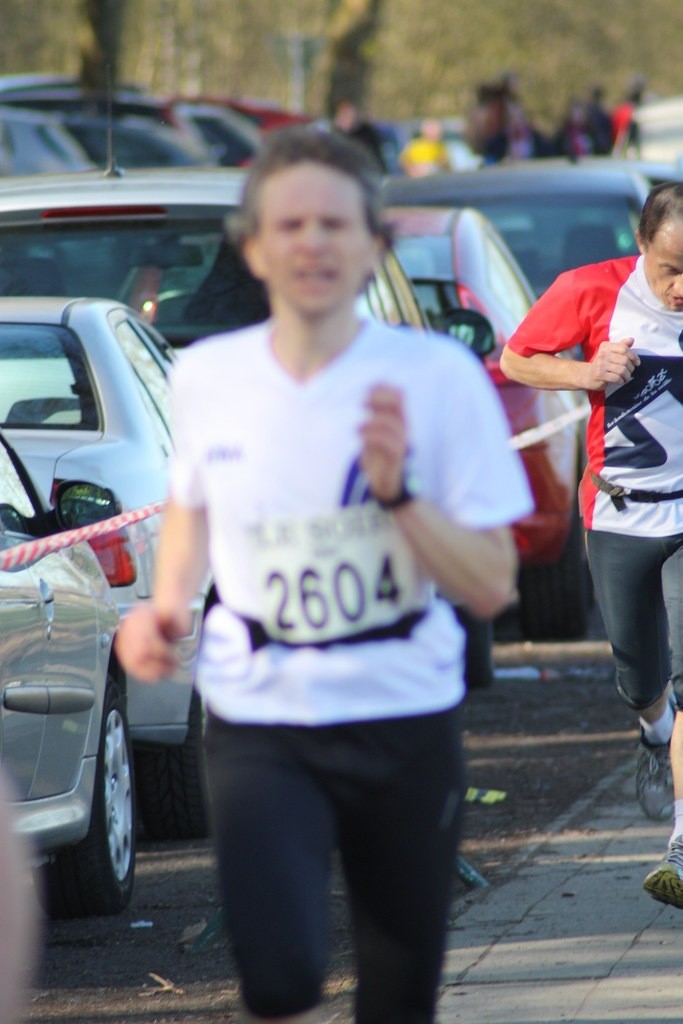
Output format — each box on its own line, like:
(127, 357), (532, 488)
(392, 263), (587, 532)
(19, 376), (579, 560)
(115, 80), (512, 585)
(376, 476), (419, 512)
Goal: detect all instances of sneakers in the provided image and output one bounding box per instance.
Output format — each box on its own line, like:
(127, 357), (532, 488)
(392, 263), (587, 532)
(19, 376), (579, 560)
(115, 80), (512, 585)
(632, 723), (674, 822)
(641, 833), (683, 909)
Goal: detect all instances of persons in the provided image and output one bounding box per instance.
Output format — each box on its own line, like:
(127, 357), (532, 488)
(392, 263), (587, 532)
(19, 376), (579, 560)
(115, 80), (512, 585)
(397, 118), (450, 178)
(114, 131), (535, 1024)
(499, 182), (683, 908)
(552, 75), (644, 156)
(463, 70), (537, 166)
(327, 102), (387, 173)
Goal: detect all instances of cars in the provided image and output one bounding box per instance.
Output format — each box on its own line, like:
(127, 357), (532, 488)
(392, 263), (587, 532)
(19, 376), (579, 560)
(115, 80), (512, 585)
(368, 205), (608, 641)
(0, 164), (504, 687)
(0, 296), (225, 836)
(0, 79), (478, 178)
(377, 162), (654, 299)
(0, 433), (141, 914)
